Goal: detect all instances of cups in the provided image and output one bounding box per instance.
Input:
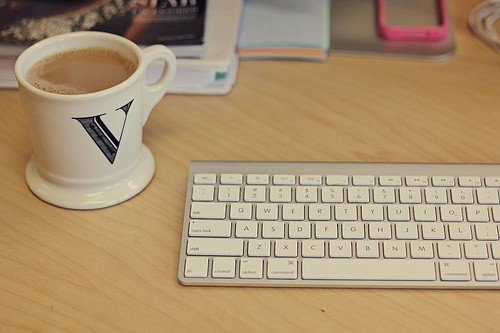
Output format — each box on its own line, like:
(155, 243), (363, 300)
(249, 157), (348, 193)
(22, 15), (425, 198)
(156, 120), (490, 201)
(12, 27), (178, 211)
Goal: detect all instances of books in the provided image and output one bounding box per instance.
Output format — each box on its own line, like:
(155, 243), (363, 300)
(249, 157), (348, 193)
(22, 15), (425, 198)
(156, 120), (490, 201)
(1, 0), (239, 94)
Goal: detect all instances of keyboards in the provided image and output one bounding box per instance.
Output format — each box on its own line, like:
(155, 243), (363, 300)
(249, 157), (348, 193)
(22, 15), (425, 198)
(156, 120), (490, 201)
(178, 160), (500, 290)
